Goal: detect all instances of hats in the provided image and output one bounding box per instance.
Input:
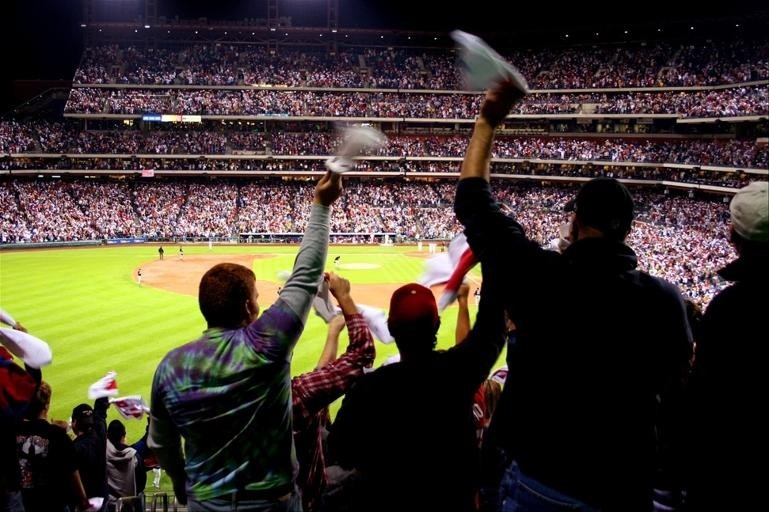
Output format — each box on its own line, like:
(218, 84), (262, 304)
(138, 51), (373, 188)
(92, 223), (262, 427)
(388, 283), (439, 329)
(73, 404), (94, 425)
(729, 181), (769, 240)
(564, 178), (635, 229)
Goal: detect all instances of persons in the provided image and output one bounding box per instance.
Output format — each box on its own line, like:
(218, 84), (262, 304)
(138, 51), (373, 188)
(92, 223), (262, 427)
(1, 42), (768, 511)
